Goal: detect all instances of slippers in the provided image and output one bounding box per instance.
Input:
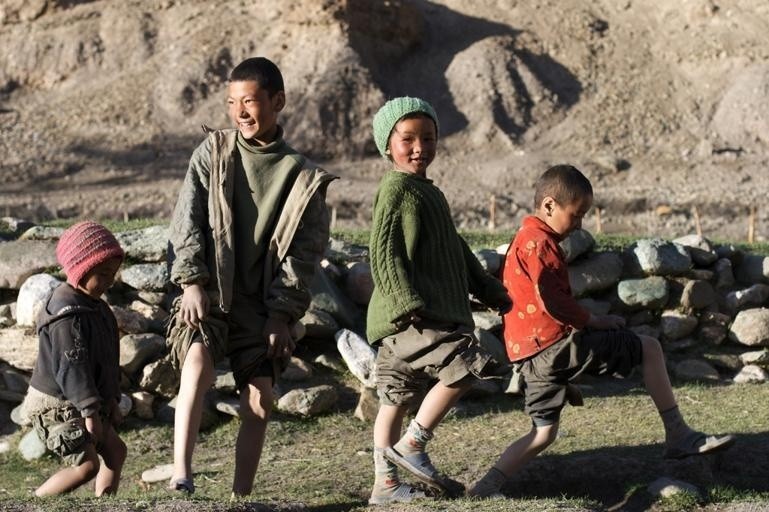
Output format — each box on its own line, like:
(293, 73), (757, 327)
(367, 478), (435, 506)
(661, 429), (739, 461)
(380, 442), (454, 491)
(167, 476), (196, 492)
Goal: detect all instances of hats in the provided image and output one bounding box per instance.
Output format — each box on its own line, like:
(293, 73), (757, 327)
(369, 93), (444, 163)
(53, 218), (131, 293)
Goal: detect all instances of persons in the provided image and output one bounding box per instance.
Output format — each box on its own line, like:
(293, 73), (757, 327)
(461, 161), (735, 500)
(168, 54), (342, 501)
(21, 220), (129, 499)
(358, 95), (516, 506)
